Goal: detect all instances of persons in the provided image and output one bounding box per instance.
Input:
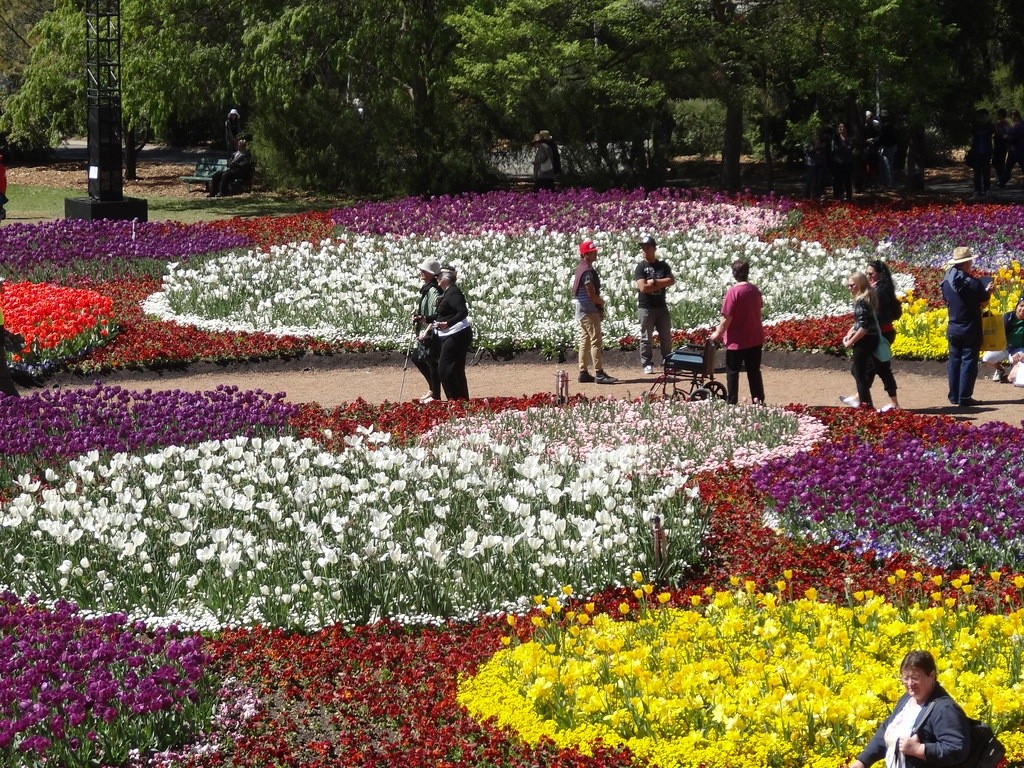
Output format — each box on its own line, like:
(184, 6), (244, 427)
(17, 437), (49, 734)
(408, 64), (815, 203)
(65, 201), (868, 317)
(531, 130), (561, 193)
(803, 109), (925, 201)
(839, 260), (902, 412)
(635, 236), (683, 375)
(411, 257), (473, 404)
(983, 297), (1024, 382)
(849, 649), (1006, 768)
(0, 277), (21, 398)
(710, 259), (765, 406)
(573, 241), (618, 384)
(0, 155), (7, 221)
(965, 108), (1024, 196)
(224, 109), (240, 159)
(939, 247), (996, 406)
(206, 140), (251, 197)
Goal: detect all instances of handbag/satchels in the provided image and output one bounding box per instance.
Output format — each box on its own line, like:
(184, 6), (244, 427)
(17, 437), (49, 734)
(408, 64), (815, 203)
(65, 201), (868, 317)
(881, 290), (903, 320)
(978, 302), (1007, 351)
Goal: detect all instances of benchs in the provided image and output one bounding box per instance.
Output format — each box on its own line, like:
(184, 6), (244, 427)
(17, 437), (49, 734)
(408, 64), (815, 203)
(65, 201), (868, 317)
(180, 148), (253, 195)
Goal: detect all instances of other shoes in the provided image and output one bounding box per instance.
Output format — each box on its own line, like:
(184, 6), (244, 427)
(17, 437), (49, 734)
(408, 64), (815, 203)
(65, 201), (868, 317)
(663, 367), (682, 375)
(421, 389), (433, 400)
(950, 397), (981, 405)
(878, 403), (901, 412)
(595, 372), (618, 384)
(874, 337), (892, 362)
(839, 396), (860, 408)
(992, 369), (1005, 381)
(420, 397), (435, 404)
(643, 366), (654, 374)
(578, 370), (595, 382)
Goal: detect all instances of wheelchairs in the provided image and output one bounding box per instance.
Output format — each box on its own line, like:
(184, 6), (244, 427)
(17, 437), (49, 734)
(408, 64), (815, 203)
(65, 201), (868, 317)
(651, 337), (727, 402)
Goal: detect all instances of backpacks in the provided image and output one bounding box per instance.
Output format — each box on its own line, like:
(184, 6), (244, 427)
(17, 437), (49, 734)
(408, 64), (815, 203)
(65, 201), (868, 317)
(909, 695), (1007, 768)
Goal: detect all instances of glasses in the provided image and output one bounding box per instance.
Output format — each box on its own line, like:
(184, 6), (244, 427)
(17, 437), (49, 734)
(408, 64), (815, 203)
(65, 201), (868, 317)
(847, 283), (855, 288)
(865, 271), (874, 276)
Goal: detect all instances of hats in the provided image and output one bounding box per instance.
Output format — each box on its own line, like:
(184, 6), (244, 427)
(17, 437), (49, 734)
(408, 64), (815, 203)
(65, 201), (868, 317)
(578, 241), (599, 254)
(948, 246), (980, 265)
(417, 257), (441, 275)
(637, 236), (658, 245)
